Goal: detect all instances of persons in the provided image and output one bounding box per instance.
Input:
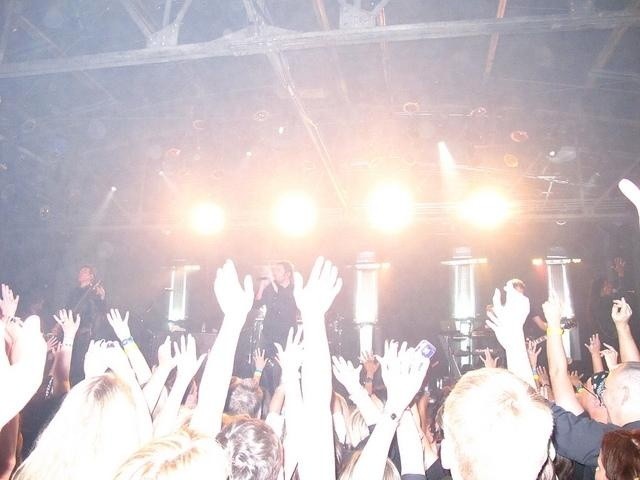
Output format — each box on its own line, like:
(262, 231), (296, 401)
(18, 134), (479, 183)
(0, 179), (640, 478)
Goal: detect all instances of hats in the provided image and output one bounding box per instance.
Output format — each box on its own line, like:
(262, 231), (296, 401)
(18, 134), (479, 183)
(504, 278), (525, 291)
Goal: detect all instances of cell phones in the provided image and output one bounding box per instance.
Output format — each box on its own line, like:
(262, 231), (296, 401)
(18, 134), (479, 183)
(413, 339), (436, 359)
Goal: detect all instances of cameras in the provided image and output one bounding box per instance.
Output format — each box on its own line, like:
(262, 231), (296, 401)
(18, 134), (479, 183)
(561, 316), (578, 330)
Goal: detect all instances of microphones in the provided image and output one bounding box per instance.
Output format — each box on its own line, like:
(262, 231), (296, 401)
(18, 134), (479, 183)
(259, 277), (271, 281)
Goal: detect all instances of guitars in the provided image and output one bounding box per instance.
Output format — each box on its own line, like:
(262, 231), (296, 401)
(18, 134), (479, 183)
(525, 317), (577, 349)
